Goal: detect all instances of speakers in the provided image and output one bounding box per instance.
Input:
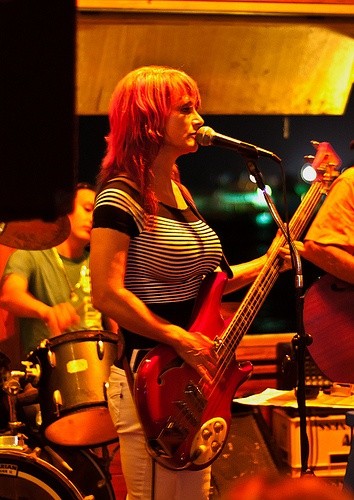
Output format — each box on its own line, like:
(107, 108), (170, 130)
(206, 410), (284, 500)
(0, 0), (78, 220)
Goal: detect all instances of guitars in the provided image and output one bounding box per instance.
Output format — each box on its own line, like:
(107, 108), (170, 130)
(132, 137), (343, 473)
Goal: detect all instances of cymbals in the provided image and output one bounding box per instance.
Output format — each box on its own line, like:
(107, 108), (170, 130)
(0, 213), (73, 251)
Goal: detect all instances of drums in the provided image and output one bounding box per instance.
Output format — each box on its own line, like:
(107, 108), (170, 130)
(0, 426), (116, 500)
(27, 329), (125, 450)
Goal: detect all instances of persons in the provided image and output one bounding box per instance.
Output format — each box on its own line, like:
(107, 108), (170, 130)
(0, 178), (124, 358)
(301, 163), (354, 387)
(84, 64), (306, 500)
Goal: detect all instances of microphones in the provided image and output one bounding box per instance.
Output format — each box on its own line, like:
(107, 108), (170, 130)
(195, 126), (282, 164)
